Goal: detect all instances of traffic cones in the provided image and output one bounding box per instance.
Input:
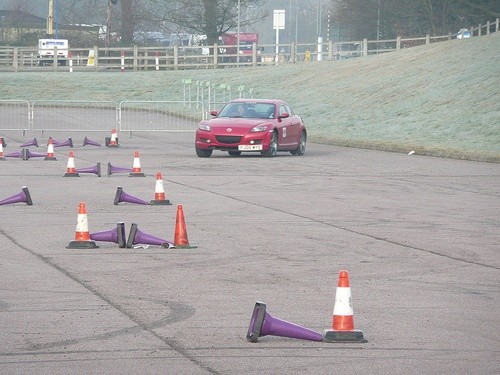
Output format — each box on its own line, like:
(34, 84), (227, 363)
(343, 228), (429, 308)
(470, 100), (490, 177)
(108, 129), (120, 148)
(82, 136), (101, 146)
(107, 162), (134, 175)
(53, 137), (74, 148)
(5, 147), (25, 160)
(129, 151), (146, 177)
(167, 204), (197, 249)
(126, 222), (175, 248)
(112, 185), (151, 206)
(47, 137), (59, 145)
(87, 221), (127, 248)
(76, 162), (102, 177)
(26, 148), (48, 161)
(148, 171), (172, 205)
(20, 138), (39, 148)
(66, 202), (101, 249)
(62, 151), (81, 178)
(322, 270), (369, 345)
(0, 186), (33, 205)
(245, 301), (324, 342)
(43, 137), (57, 162)
(0, 137), (7, 160)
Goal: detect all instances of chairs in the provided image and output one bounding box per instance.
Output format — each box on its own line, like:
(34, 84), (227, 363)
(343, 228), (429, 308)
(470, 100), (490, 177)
(267, 107), (274, 115)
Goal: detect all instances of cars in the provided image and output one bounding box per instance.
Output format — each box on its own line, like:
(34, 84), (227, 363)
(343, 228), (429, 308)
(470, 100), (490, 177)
(191, 99), (308, 158)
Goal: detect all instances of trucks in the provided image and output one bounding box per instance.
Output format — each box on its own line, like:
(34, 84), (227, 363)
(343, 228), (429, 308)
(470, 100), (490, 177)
(107, 30), (265, 66)
(35, 39), (69, 65)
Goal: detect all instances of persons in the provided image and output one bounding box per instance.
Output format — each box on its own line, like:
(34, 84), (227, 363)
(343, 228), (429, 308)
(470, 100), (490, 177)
(236, 104), (250, 117)
